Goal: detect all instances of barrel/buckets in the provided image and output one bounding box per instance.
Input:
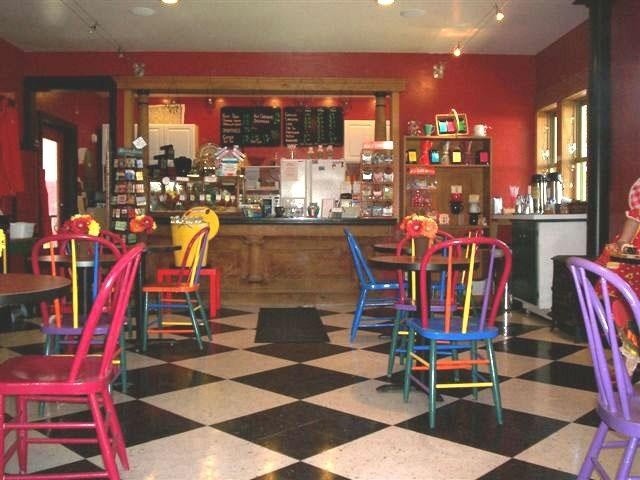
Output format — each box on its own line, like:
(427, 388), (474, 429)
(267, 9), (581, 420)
(171, 222), (210, 270)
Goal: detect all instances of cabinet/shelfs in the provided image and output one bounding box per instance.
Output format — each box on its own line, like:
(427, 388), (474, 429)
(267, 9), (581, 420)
(403, 135), (493, 306)
(509, 220), (588, 322)
(132, 122), (199, 168)
(343, 119), (391, 162)
(546, 255), (600, 344)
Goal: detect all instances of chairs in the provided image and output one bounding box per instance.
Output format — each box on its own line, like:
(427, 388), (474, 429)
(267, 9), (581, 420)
(387, 230), (462, 382)
(343, 227), (408, 343)
(404, 237), (512, 429)
(142, 226), (213, 352)
(32, 230), (133, 418)
(0, 242), (145, 480)
(566, 257), (640, 480)
(431, 227), (484, 305)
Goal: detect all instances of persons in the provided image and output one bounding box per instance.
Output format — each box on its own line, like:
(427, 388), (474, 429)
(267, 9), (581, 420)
(592, 177), (640, 377)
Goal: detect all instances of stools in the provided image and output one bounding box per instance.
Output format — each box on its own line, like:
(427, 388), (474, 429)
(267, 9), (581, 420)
(157, 268), (221, 318)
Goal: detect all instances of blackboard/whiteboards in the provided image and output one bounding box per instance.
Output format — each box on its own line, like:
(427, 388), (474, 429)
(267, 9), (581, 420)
(282, 108), (342, 146)
(220, 105), (281, 147)
